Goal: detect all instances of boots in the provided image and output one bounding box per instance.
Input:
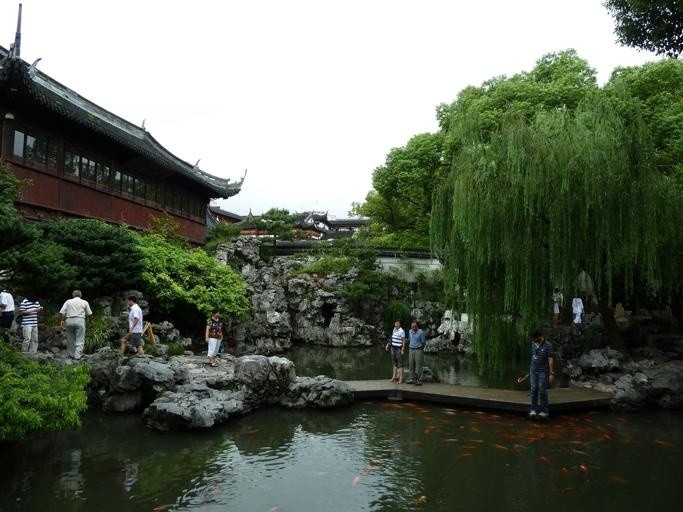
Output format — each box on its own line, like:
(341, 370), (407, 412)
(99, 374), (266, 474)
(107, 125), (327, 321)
(390, 367), (398, 382)
(396, 367), (403, 384)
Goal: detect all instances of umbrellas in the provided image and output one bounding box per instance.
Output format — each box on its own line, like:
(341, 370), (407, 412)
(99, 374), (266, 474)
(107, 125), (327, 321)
(517, 374), (529, 383)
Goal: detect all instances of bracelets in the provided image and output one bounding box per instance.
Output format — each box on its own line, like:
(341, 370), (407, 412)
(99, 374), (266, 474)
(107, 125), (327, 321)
(550, 372), (554, 375)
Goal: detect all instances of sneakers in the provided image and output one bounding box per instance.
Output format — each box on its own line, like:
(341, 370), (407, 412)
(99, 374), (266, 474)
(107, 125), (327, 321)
(538, 413), (548, 418)
(529, 411), (538, 417)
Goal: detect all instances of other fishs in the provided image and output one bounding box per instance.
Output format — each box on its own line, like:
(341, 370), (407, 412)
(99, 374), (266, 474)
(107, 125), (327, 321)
(239, 425), (257, 438)
(351, 401), (679, 505)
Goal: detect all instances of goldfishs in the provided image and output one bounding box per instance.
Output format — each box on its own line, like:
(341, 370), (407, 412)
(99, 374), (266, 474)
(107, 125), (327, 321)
(351, 401), (679, 505)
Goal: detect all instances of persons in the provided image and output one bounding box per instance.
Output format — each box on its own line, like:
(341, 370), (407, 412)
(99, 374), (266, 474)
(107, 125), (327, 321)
(59, 290), (93, 360)
(0, 286), (15, 343)
(385, 320), (406, 384)
(205, 309), (224, 367)
(529, 330), (555, 417)
(407, 320), (426, 385)
(572, 297), (585, 324)
(120, 296), (144, 356)
(552, 288), (563, 319)
(19, 292), (44, 352)
